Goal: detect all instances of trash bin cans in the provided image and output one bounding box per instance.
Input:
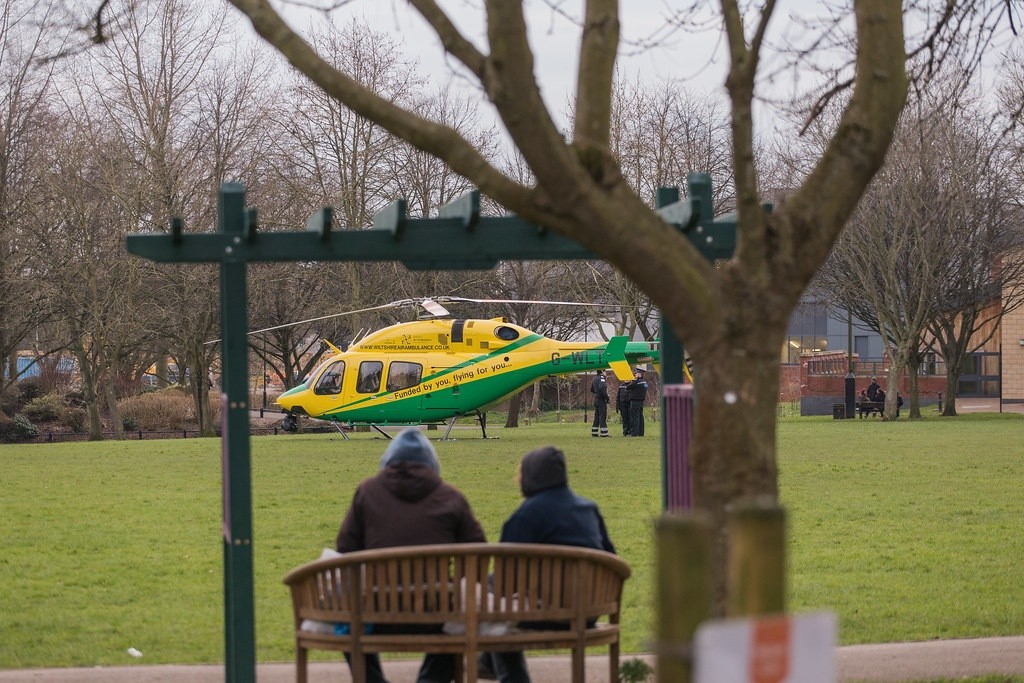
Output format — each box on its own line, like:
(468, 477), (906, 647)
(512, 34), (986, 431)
(832, 402), (847, 419)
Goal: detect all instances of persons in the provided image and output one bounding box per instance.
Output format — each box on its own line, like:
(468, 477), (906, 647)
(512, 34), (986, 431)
(335, 428), (490, 683)
(616, 380), (632, 436)
(490, 446), (616, 683)
(626, 368), (647, 436)
(591, 368), (613, 437)
(859, 389), (870, 418)
(875, 389), (885, 417)
(896, 392), (903, 417)
(867, 377), (880, 418)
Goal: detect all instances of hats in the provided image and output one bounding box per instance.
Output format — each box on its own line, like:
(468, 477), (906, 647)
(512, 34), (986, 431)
(381, 427), (439, 466)
(636, 368), (646, 374)
(522, 446), (567, 494)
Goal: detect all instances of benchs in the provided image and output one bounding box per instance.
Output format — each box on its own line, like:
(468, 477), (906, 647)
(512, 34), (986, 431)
(281, 542), (633, 683)
(855, 402), (900, 420)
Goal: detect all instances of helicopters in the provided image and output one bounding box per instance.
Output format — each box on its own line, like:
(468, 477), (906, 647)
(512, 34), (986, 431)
(202, 296), (696, 443)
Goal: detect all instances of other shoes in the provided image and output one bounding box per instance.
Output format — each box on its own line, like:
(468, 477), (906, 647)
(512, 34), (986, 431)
(474, 657), (498, 681)
